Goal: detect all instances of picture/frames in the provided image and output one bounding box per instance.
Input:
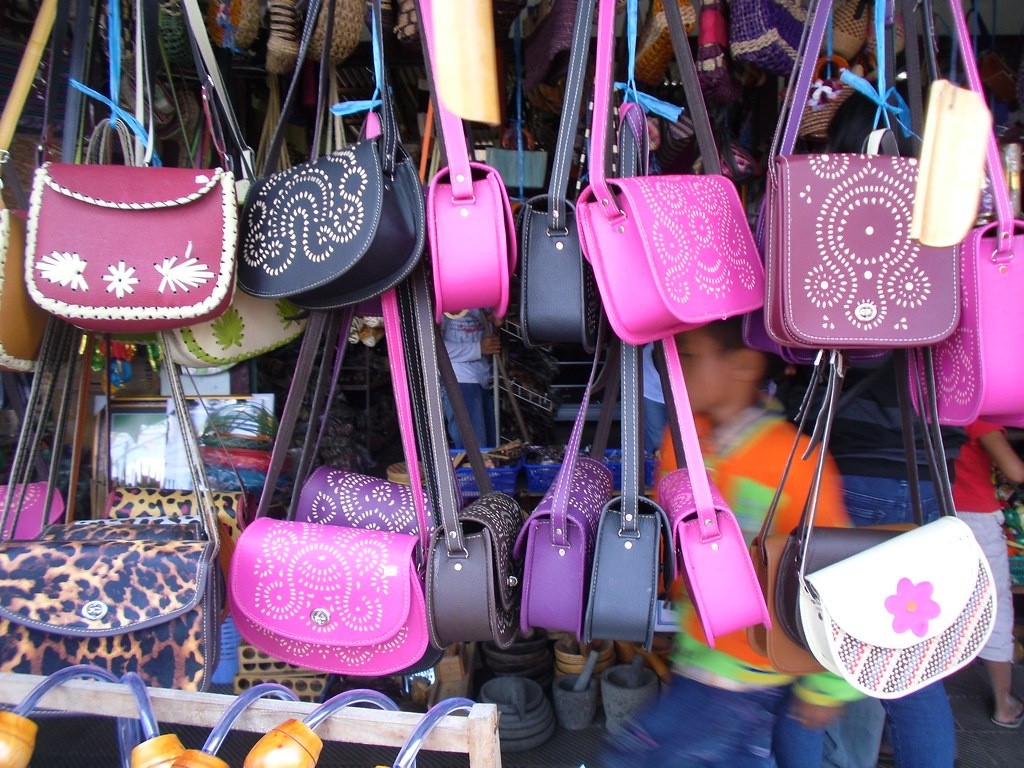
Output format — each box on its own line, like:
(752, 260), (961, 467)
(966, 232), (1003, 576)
(91, 393), (276, 517)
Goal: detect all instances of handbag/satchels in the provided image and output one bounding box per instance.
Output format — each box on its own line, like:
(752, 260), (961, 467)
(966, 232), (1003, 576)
(20, 158), (239, 335)
(657, 466), (775, 649)
(508, 454), (614, 646)
(0, 479), (254, 625)
(583, 494), (677, 653)
(575, 171), (767, 345)
(515, 192), (611, 352)
(239, 134), (427, 313)
(905, 219), (1024, 428)
(739, 195), (899, 366)
(798, 515), (997, 700)
(774, 524), (911, 653)
(156, 176), (310, 368)
(225, 514), (430, 676)
(423, 491), (527, 653)
(97, 0), (909, 138)
(0, 208), (55, 374)
(423, 162), (519, 324)
(759, 149), (960, 353)
(746, 521), (920, 675)
(294, 464), (437, 557)
(389, 645), (444, 676)
(0, 538), (221, 692)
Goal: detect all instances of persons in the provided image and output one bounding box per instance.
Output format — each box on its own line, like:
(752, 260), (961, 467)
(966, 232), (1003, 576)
(599, 317), (857, 768)
(774, 360), (956, 768)
(950, 420), (1024, 727)
(437, 309), (501, 449)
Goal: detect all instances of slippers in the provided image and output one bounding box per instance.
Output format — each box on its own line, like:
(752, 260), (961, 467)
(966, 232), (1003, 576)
(991, 692), (1024, 728)
